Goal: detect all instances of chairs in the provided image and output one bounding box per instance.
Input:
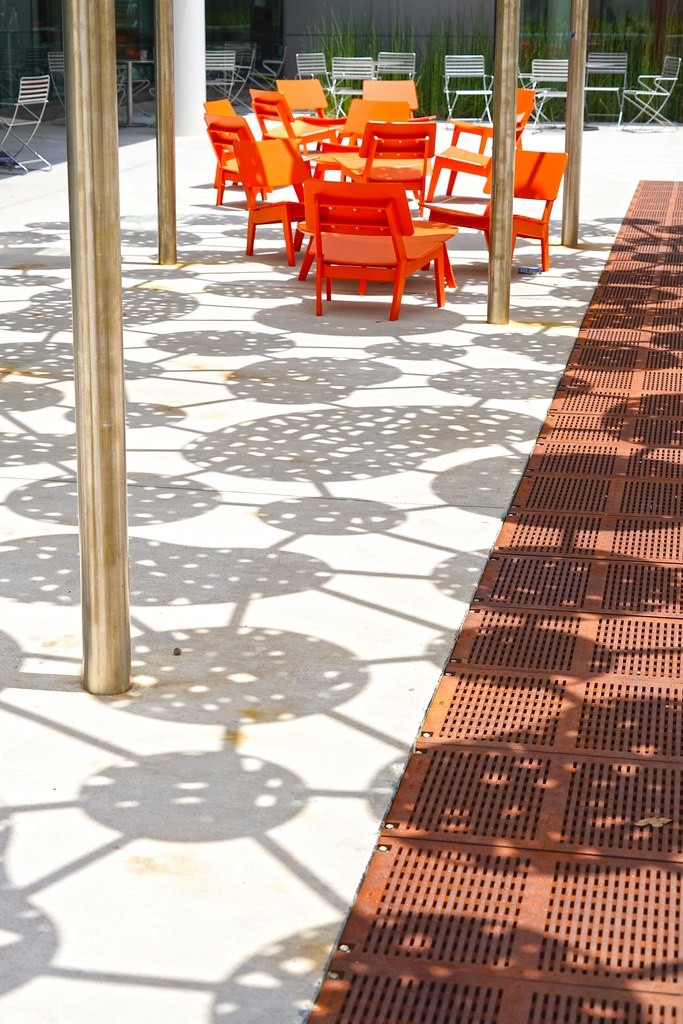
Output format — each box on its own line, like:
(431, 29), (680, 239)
(585, 52), (682, 132)
(205, 41), (570, 321)
(0, 74), (52, 174)
(12, 47), (51, 98)
(47, 52), (63, 108)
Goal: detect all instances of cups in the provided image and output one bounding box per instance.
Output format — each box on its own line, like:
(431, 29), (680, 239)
(140, 50), (148, 61)
